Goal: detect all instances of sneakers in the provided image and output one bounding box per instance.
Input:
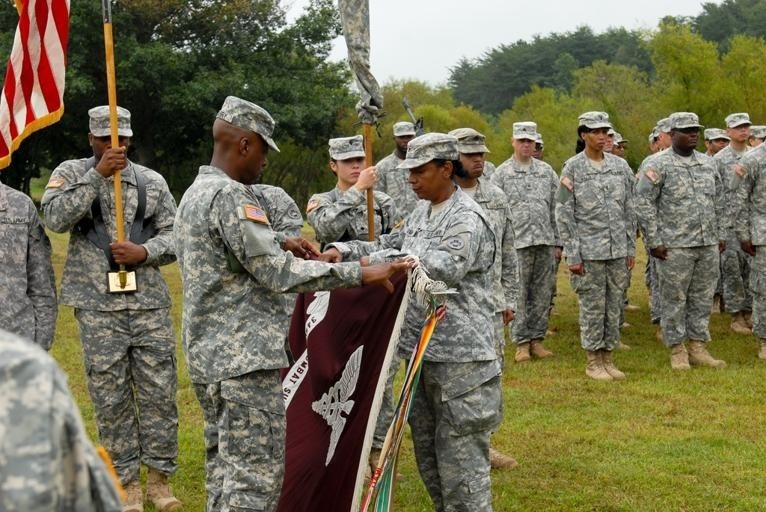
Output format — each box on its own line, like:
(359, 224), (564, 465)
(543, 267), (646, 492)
(624, 304), (635, 311)
(489, 448), (516, 472)
(619, 322), (629, 328)
(617, 340), (630, 351)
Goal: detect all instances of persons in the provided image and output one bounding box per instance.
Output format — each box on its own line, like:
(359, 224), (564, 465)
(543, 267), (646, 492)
(42, 106), (178, 511)
(319, 133), (506, 512)
(2, 329), (120, 512)
(174, 94), (413, 512)
(483, 112), (766, 382)
(375, 121), (419, 219)
(250, 185), (303, 248)
(0, 182), (57, 352)
(449, 128), (522, 472)
(308, 134), (394, 248)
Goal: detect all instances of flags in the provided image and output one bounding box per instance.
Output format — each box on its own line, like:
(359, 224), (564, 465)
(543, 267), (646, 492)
(0, 0), (71, 166)
(280, 260), (413, 510)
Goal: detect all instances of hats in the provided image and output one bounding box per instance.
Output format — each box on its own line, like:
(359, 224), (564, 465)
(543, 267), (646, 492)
(88, 106), (134, 138)
(449, 128), (490, 154)
(395, 133), (460, 169)
(579, 111), (628, 146)
(393, 122), (416, 136)
(513, 121), (544, 144)
(649, 111), (765, 144)
(328, 135), (366, 160)
(216, 96), (280, 153)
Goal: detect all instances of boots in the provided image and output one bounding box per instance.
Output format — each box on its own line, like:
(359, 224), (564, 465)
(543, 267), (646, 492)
(587, 348), (625, 382)
(758, 338), (766, 360)
(711, 296), (752, 333)
(122, 479), (143, 511)
(370, 448), (404, 481)
(670, 338), (725, 371)
(515, 340), (553, 362)
(147, 470), (180, 512)
